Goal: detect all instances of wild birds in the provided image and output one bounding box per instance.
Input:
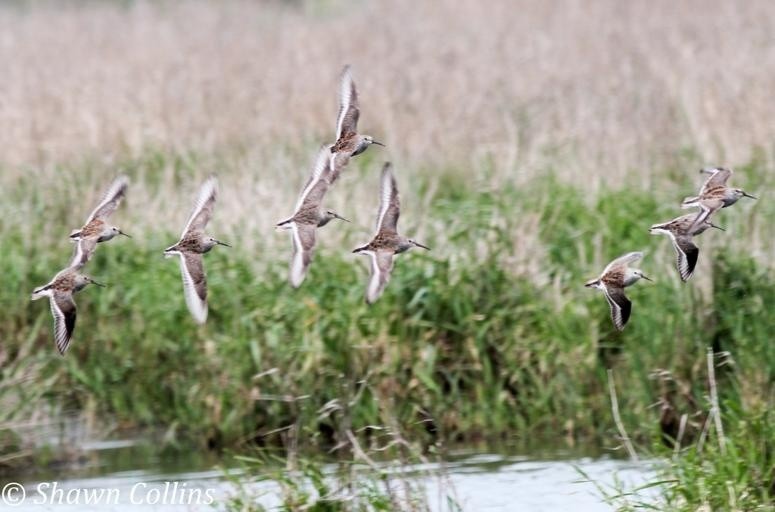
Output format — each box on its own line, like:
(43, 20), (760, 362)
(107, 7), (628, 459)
(647, 212), (726, 282)
(681, 167), (759, 236)
(69, 173), (131, 272)
(29, 255), (106, 357)
(351, 161), (431, 304)
(584, 251), (653, 333)
(163, 172), (233, 327)
(323, 64), (387, 178)
(274, 146), (352, 291)
